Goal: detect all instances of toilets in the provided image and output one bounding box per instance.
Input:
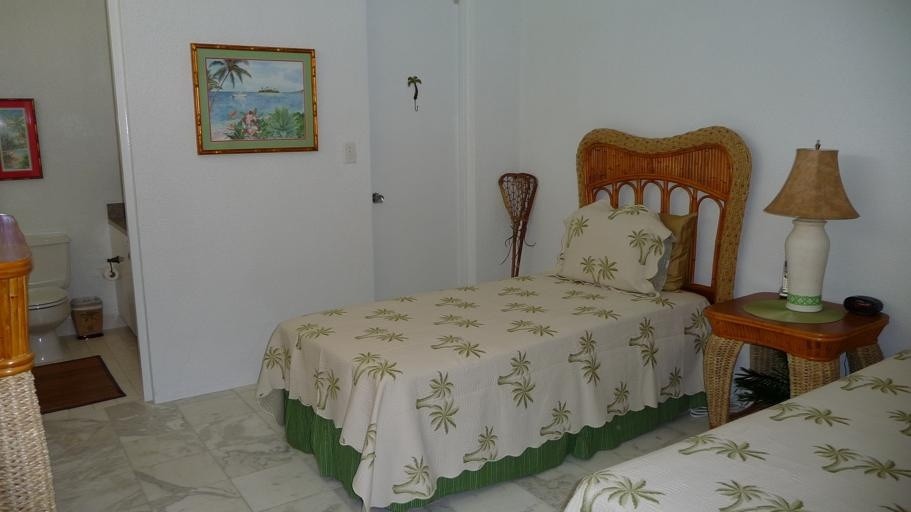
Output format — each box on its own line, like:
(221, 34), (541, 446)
(24, 233), (73, 361)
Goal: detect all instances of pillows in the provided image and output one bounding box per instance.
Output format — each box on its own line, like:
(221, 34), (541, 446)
(545, 200), (697, 299)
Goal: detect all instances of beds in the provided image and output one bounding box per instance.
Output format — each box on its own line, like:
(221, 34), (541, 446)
(564, 348), (911, 512)
(280, 127), (752, 512)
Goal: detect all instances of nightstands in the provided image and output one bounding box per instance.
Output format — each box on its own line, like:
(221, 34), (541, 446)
(702, 293), (889, 429)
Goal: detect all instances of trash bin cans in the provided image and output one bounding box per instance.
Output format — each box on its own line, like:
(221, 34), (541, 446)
(70, 297), (103, 340)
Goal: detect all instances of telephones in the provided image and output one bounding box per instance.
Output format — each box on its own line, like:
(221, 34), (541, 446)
(776, 262), (790, 300)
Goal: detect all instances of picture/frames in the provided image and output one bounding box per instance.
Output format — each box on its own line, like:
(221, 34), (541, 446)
(190, 42), (319, 155)
(0, 98), (44, 181)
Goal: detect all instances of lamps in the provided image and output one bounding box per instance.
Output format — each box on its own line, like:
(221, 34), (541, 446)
(763, 140), (860, 313)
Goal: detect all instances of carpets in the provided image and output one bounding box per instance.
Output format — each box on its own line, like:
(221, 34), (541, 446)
(32, 355), (126, 415)
